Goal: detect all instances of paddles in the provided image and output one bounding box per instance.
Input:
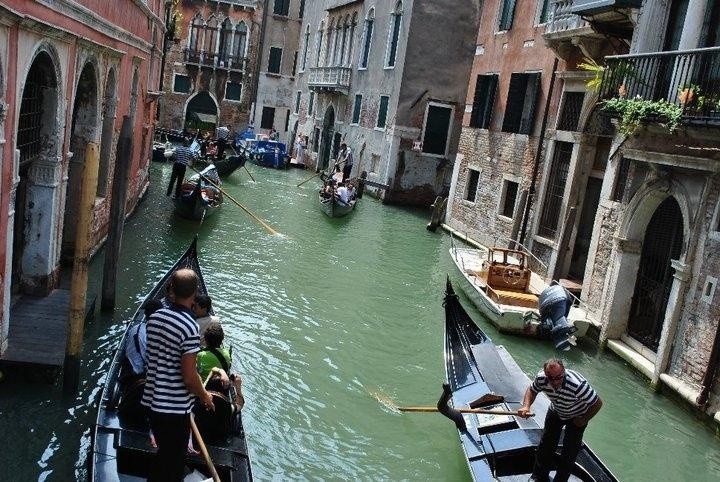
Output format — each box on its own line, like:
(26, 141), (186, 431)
(187, 164), (278, 235)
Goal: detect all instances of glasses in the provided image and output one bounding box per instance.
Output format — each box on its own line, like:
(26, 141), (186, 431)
(547, 375), (564, 381)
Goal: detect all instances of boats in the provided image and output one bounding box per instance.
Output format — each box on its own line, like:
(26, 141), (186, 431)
(153, 125), (292, 221)
(93, 234), (253, 482)
(319, 167), (357, 217)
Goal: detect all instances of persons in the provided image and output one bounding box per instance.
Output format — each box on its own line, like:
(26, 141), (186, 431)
(515, 359), (602, 482)
(216, 124), (231, 159)
(337, 143), (357, 179)
(295, 132), (306, 165)
(268, 129), (279, 141)
(166, 136), (198, 200)
(322, 178), (358, 209)
(123, 282), (245, 419)
(205, 143), (218, 159)
(142, 267), (215, 482)
